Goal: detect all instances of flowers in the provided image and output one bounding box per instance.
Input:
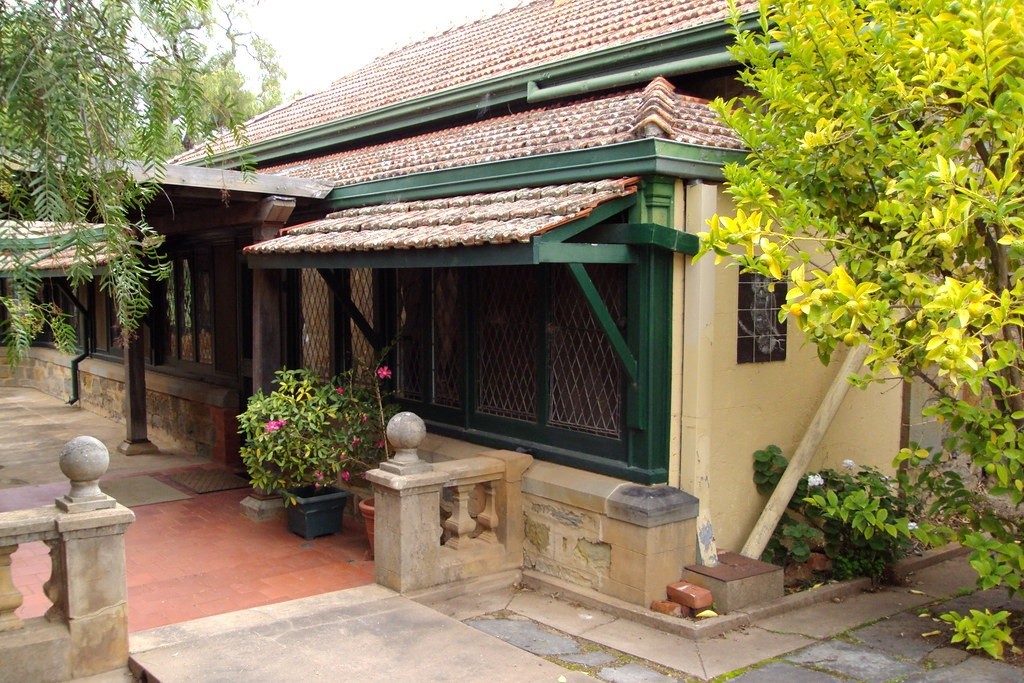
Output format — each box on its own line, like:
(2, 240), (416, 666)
(237, 330), (409, 502)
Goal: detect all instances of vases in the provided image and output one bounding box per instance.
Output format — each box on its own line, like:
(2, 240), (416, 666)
(283, 483), (351, 541)
(358, 496), (375, 557)
